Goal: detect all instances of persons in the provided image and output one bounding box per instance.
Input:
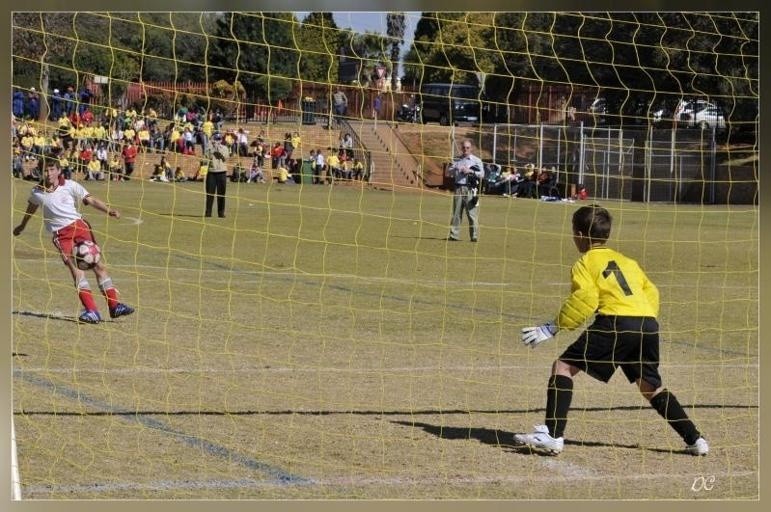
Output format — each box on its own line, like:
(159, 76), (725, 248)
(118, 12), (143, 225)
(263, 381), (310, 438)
(442, 139), (484, 242)
(482, 162), (557, 199)
(513, 203), (709, 458)
(12, 153), (135, 324)
(204, 133), (229, 218)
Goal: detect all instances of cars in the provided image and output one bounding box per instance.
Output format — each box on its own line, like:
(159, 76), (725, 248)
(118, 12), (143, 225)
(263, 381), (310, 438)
(396, 101), (414, 122)
(575, 92), (732, 132)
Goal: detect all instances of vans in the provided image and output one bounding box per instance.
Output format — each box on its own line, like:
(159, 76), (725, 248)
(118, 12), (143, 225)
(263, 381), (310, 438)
(416, 81), (482, 127)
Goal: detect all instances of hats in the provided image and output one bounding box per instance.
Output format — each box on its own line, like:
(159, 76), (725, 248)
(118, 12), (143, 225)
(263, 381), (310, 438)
(213, 134), (222, 140)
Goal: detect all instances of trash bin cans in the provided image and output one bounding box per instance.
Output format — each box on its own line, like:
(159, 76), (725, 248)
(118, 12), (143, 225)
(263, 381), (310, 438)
(296, 159), (314, 184)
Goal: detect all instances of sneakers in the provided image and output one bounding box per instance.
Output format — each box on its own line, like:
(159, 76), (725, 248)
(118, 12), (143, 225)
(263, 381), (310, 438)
(109, 303), (135, 318)
(686, 436), (709, 456)
(513, 425), (564, 457)
(79, 311), (101, 324)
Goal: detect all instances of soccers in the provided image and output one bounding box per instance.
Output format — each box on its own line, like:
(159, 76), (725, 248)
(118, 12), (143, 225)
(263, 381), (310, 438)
(71, 241), (101, 270)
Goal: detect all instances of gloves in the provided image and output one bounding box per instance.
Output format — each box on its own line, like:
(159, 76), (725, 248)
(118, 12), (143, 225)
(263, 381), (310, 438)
(520, 320), (558, 349)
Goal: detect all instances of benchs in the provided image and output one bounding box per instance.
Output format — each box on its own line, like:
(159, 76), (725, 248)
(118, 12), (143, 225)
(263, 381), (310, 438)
(26, 122), (358, 185)
(531, 177), (562, 200)
